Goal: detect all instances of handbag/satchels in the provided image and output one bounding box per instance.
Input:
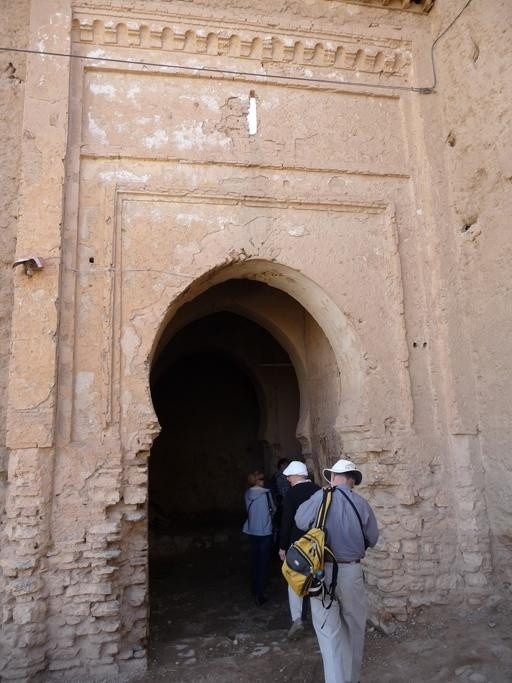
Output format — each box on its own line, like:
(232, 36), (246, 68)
(272, 525), (280, 545)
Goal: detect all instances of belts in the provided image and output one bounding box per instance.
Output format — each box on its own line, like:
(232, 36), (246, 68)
(326, 560), (360, 564)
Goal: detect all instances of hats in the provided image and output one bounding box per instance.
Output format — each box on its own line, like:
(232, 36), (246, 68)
(282, 460), (308, 476)
(323, 459), (362, 486)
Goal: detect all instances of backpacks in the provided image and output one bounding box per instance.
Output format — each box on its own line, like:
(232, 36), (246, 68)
(281, 486), (338, 610)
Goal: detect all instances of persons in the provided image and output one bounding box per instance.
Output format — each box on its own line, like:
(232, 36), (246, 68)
(274, 458), (322, 640)
(243, 470), (277, 606)
(295, 459), (378, 683)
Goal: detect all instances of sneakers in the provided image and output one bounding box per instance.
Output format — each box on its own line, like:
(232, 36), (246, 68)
(286, 618), (305, 639)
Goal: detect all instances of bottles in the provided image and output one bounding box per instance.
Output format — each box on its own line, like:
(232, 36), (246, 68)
(310, 571), (324, 585)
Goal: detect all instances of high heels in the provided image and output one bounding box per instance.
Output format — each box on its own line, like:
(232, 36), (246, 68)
(256, 592), (270, 605)
